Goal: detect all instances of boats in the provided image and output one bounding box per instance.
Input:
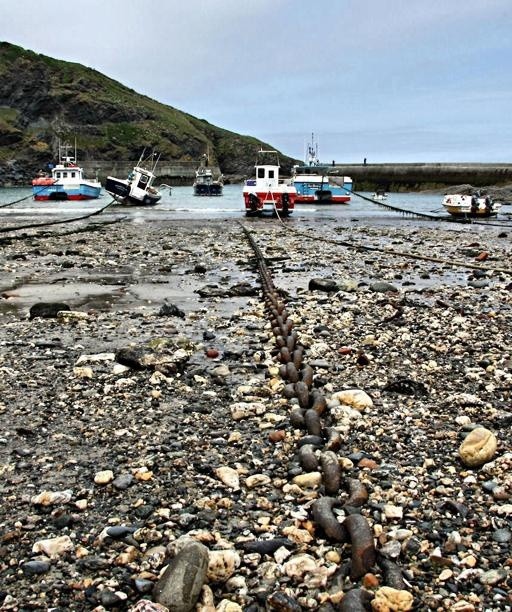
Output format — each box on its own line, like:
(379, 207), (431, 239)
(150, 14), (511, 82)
(32, 138), (102, 202)
(243, 149), (297, 215)
(290, 164), (353, 204)
(442, 193), (502, 218)
(193, 165), (223, 196)
(105, 150), (162, 206)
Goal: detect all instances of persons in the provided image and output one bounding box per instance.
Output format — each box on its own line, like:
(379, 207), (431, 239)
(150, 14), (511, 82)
(471, 194), (480, 218)
(203, 153), (208, 166)
(247, 192), (260, 212)
(485, 195), (493, 218)
(281, 192), (289, 215)
